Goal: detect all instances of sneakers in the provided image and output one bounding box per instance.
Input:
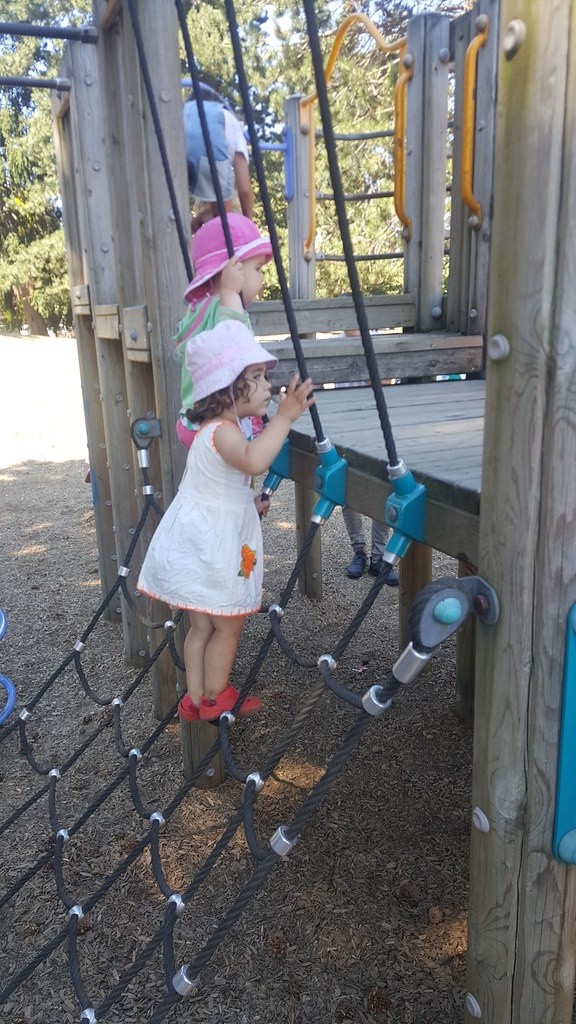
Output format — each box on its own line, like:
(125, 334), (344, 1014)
(346, 553), (368, 577)
(367, 557), (400, 586)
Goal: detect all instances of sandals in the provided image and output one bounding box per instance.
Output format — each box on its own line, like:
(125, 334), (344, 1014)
(199, 686), (263, 721)
(177, 694), (200, 721)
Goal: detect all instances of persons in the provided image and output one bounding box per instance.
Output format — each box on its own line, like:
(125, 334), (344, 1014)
(178, 213), (274, 447)
(136, 319), (315, 722)
(330, 292), (398, 587)
(184, 73), (254, 233)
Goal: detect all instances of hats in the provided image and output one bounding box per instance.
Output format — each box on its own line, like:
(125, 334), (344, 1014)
(185, 319), (278, 404)
(183, 212), (273, 305)
